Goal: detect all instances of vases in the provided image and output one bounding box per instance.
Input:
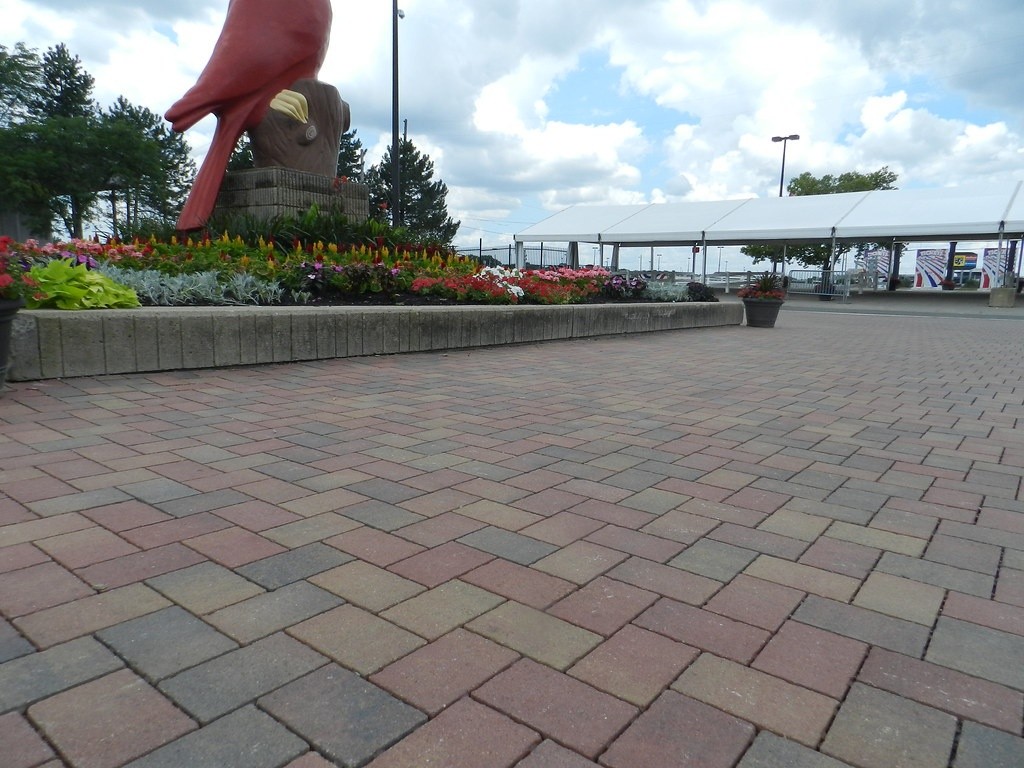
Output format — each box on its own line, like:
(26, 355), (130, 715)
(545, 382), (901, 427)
(741, 299), (785, 328)
(942, 286), (954, 290)
(889, 284), (896, 291)
(0, 302), (25, 388)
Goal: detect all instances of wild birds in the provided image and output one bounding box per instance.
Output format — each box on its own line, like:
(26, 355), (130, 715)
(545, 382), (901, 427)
(164, 1), (332, 231)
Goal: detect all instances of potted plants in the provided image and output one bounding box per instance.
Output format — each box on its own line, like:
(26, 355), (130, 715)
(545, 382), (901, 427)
(815, 283), (835, 301)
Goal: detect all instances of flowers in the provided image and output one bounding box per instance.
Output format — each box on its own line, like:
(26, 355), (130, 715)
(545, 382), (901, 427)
(941, 279), (955, 286)
(0, 234), (49, 302)
(738, 270), (784, 300)
(883, 276), (900, 284)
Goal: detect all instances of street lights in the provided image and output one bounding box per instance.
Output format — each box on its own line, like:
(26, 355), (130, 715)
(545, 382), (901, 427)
(772, 134), (800, 198)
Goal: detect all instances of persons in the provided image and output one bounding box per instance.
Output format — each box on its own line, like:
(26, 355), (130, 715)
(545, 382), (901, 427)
(550, 262), (594, 275)
